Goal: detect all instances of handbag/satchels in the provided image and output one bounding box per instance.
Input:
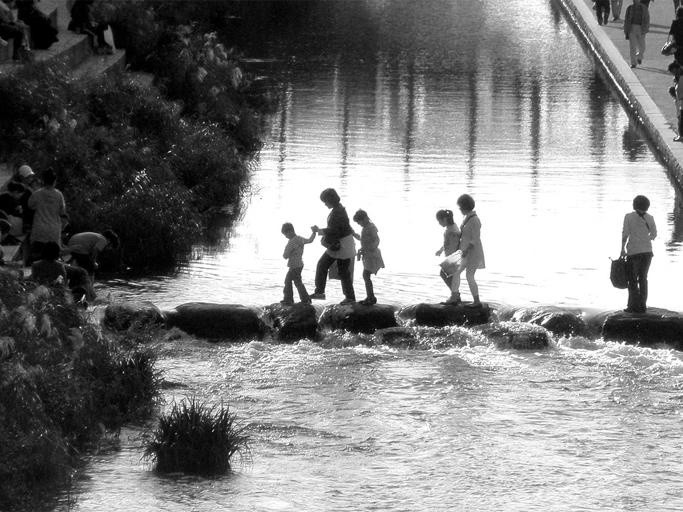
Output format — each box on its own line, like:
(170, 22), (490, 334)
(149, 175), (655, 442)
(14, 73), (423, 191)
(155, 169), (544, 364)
(609, 253), (630, 289)
(329, 257), (354, 281)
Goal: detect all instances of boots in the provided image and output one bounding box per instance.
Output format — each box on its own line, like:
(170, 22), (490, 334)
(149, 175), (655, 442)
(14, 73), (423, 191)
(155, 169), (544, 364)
(440, 292), (483, 309)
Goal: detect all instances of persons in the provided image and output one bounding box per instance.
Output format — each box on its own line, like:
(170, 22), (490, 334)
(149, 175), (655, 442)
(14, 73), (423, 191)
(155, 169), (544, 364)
(308, 188), (356, 307)
(67, 1), (113, 57)
(0, 0), (28, 61)
(592, 0), (681, 143)
(444, 194), (483, 309)
(350, 210), (384, 305)
(280, 221), (316, 306)
(619, 195), (657, 313)
(433, 208), (461, 305)
(0, 163), (118, 309)
(16, 0), (59, 50)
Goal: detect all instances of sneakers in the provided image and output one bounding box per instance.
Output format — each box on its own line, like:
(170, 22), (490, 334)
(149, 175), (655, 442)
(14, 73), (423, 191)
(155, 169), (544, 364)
(280, 299), (294, 304)
(359, 296), (376, 305)
(340, 298), (357, 305)
(308, 292), (326, 300)
(299, 298), (312, 304)
